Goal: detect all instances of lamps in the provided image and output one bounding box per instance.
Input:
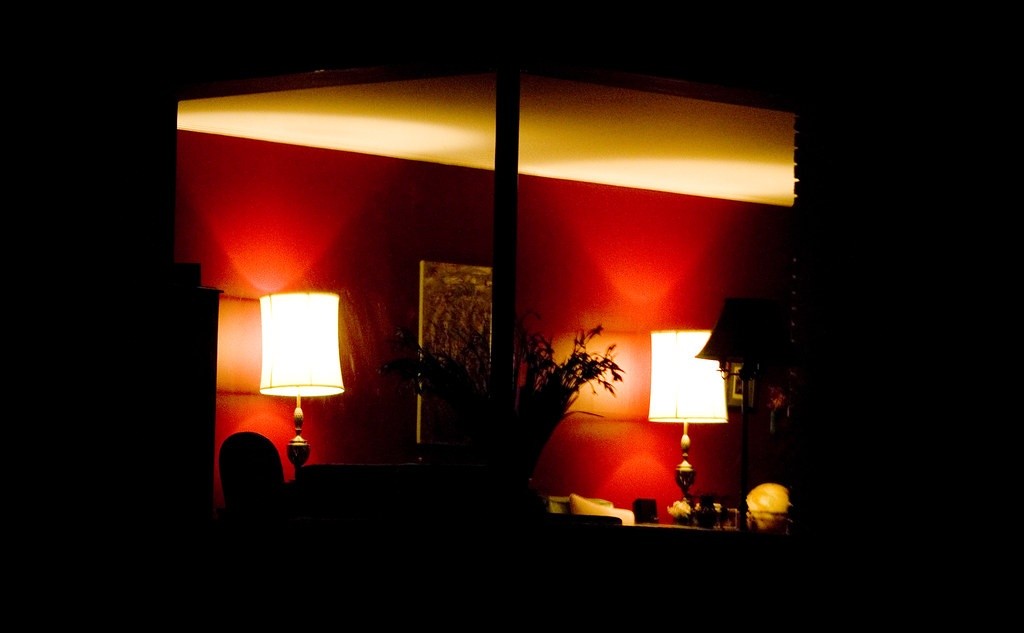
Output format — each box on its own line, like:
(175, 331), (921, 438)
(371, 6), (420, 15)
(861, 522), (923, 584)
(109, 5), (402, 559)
(699, 295), (799, 530)
(259, 290), (346, 478)
(648, 329), (729, 526)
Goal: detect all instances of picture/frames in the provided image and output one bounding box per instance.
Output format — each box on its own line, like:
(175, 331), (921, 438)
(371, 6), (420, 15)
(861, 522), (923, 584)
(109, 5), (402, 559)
(416, 258), (493, 445)
(727, 362), (753, 409)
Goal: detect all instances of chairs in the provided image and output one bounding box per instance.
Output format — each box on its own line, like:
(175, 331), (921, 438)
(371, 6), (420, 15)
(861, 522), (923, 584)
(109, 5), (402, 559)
(219, 431), (285, 512)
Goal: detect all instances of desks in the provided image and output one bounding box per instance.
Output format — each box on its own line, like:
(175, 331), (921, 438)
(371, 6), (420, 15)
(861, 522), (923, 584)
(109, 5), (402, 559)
(543, 520), (798, 633)
(295, 463), (490, 531)
